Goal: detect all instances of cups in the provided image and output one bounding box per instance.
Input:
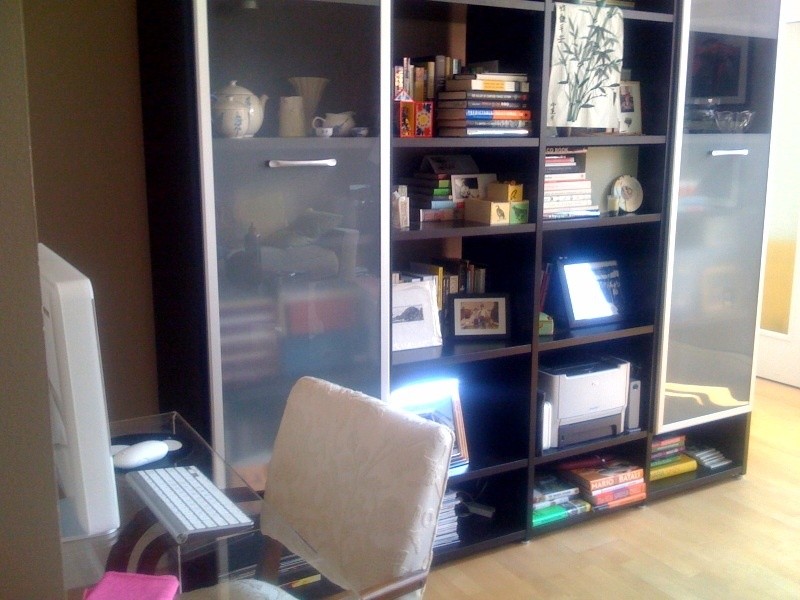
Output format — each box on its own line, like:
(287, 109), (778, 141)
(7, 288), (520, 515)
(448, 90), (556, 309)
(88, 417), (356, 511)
(352, 128), (369, 136)
(279, 96), (307, 138)
(315, 127), (333, 137)
(606, 194), (619, 216)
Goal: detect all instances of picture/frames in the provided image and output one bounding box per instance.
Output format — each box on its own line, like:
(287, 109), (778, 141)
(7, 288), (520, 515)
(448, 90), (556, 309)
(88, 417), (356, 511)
(556, 252), (636, 329)
(449, 171), (500, 204)
(391, 279), (443, 352)
(447, 292), (512, 342)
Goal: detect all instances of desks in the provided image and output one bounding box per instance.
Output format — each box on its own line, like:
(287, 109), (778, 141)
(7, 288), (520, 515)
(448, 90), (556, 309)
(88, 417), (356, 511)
(50, 410), (365, 600)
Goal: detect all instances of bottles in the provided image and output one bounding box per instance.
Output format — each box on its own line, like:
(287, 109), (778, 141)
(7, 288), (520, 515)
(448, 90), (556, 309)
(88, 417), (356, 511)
(243, 225), (262, 272)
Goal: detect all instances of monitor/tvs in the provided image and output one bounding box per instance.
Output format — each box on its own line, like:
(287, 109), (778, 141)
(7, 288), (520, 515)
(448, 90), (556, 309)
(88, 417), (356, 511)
(36, 241), (121, 544)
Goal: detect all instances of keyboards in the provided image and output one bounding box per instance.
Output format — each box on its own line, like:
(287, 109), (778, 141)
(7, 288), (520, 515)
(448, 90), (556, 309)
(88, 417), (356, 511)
(125, 465), (254, 543)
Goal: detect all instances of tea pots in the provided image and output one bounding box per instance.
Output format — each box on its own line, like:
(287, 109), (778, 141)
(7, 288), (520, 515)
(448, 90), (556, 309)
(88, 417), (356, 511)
(210, 81), (269, 138)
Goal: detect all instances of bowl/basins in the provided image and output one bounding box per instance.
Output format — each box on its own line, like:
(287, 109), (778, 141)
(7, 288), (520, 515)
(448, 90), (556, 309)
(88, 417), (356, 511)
(714, 112), (754, 133)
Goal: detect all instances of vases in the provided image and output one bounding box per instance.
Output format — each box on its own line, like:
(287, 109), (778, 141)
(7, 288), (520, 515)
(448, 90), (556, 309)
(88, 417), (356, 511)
(713, 109), (756, 132)
(279, 95), (306, 139)
(287, 76), (328, 137)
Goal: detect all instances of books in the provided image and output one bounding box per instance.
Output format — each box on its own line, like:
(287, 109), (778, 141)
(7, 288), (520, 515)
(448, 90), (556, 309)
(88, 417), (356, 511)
(227, 540), (323, 599)
(398, 170), (489, 227)
(388, 54), (535, 140)
(430, 430), (741, 552)
(542, 146), (602, 220)
(392, 254), (486, 349)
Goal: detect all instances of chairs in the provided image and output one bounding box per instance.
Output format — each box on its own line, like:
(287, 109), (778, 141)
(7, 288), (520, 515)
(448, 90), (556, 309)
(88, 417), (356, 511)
(170, 376), (457, 600)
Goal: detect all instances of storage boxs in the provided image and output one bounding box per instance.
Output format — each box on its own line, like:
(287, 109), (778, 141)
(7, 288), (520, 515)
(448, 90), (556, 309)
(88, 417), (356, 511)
(214, 272), (373, 393)
(394, 100), (434, 138)
(464, 182), (529, 226)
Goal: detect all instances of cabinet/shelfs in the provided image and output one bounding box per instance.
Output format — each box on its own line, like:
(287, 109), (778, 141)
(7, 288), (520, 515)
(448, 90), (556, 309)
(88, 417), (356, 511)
(137, 0), (783, 600)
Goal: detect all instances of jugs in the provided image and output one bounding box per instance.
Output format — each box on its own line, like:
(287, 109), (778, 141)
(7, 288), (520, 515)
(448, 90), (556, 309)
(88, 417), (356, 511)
(312, 111), (356, 136)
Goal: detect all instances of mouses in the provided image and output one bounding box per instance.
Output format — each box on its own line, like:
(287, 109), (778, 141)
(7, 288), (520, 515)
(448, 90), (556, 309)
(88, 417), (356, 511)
(114, 440), (168, 470)
